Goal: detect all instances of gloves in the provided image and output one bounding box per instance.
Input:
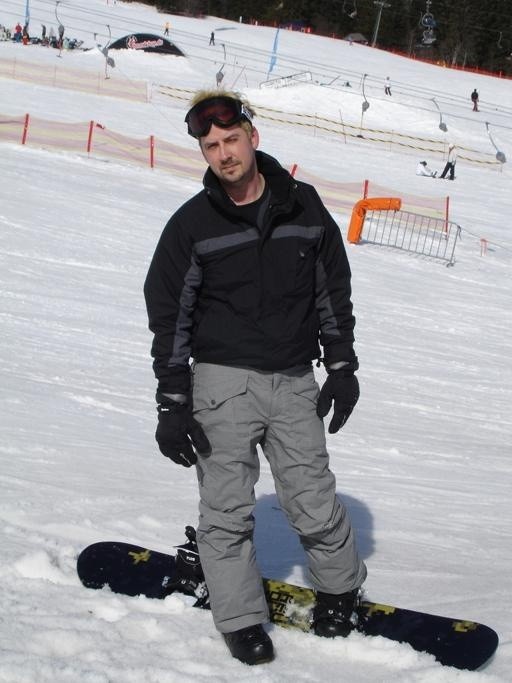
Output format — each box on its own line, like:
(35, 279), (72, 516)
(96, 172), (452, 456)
(153, 389), (212, 469)
(315, 355), (362, 434)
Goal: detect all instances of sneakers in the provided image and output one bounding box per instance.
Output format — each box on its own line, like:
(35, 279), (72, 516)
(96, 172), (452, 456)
(222, 623), (274, 666)
(311, 586), (363, 637)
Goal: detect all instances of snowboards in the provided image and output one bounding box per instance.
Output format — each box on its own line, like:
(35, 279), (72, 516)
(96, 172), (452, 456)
(77, 541), (498, 672)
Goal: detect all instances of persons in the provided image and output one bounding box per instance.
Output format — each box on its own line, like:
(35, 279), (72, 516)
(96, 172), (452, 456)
(415, 161), (437, 178)
(209, 31), (215, 46)
(144, 85), (369, 665)
(384, 76), (391, 96)
(439, 143), (457, 180)
(14, 23), (77, 49)
(471, 89), (479, 111)
(164, 21), (169, 36)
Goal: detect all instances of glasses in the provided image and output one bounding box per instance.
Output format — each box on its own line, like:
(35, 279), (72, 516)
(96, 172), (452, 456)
(183, 95), (253, 138)
